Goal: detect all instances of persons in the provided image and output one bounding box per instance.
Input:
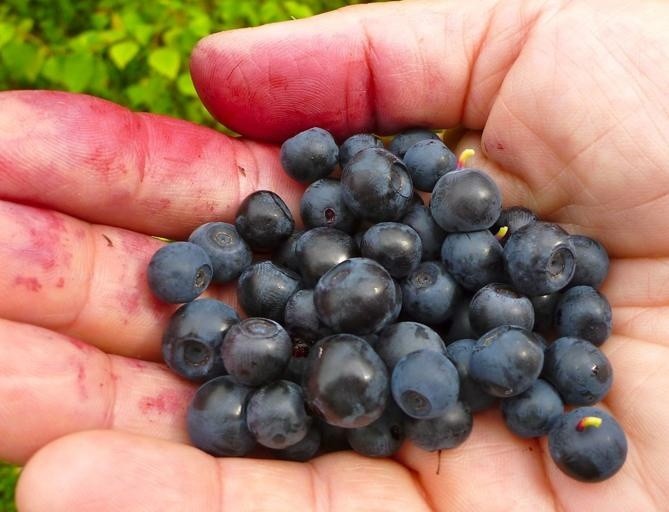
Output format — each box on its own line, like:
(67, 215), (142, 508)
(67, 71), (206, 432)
(0, 0), (669, 512)
